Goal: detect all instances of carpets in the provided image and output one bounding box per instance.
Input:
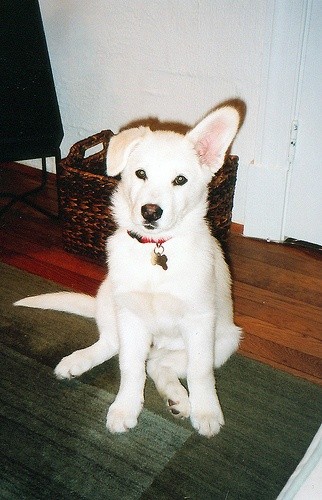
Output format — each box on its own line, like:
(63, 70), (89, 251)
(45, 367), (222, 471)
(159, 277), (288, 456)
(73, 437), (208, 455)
(1, 263), (322, 500)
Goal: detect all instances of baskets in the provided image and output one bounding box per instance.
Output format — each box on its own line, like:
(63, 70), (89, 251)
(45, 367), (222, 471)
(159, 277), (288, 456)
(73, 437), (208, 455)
(55, 130), (241, 266)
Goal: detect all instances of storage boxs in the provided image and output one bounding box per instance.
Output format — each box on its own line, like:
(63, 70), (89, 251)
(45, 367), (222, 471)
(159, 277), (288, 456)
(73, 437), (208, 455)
(56, 129), (238, 267)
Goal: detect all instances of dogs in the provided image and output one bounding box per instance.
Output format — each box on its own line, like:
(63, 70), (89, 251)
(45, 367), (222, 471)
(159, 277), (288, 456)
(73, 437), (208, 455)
(11, 105), (245, 439)
